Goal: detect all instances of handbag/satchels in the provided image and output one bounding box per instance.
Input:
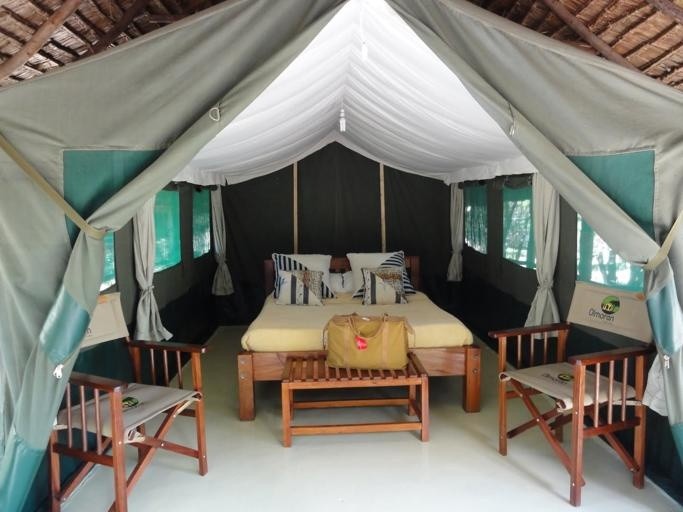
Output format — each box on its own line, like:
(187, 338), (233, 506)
(327, 313), (410, 371)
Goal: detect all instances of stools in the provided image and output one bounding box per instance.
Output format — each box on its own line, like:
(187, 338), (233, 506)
(487, 322), (649, 507)
(42, 339), (209, 512)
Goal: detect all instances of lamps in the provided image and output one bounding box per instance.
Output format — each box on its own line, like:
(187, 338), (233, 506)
(339, 109), (346, 135)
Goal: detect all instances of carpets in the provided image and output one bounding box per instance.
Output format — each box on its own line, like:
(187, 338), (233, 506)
(57, 320), (680, 512)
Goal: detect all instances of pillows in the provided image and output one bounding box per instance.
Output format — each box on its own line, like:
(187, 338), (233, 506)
(344, 251), (415, 307)
(272, 249), (341, 308)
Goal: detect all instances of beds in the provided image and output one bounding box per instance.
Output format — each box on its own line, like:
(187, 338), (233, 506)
(236, 254), (484, 423)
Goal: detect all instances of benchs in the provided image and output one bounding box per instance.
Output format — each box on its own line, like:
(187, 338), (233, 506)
(278, 350), (429, 446)
